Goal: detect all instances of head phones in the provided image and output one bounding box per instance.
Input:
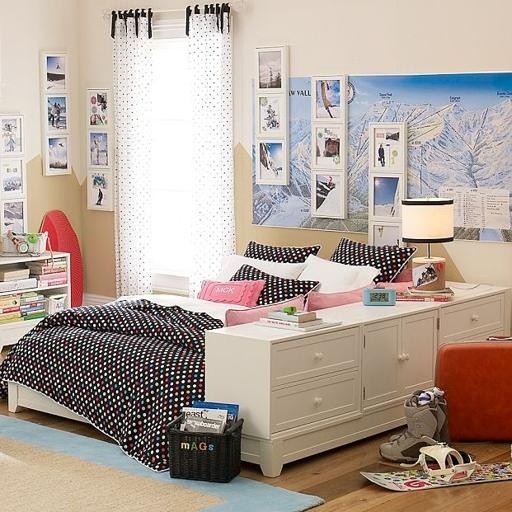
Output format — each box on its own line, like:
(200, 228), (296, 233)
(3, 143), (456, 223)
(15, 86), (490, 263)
(7, 230), (29, 254)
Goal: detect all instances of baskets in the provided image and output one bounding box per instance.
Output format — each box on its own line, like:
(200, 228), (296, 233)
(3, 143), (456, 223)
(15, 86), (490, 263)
(1, 231), (49, 257)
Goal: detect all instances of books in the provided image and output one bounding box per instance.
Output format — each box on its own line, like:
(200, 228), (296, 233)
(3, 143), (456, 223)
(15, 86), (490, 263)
(1, 258), (66, 325)
(255, 310), (341, 332)
(376, 282), (454, 302)
(179, 400), (239, 434)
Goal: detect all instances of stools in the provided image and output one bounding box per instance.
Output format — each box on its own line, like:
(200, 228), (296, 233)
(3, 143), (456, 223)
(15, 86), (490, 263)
(434, 336), (512, 442)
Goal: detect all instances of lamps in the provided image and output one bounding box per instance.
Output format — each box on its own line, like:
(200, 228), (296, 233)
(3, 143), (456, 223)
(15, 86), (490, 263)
(401, 197), (455, 291)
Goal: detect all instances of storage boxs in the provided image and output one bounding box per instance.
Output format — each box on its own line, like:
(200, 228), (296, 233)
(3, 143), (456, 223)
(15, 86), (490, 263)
(2, 230), (48, 254)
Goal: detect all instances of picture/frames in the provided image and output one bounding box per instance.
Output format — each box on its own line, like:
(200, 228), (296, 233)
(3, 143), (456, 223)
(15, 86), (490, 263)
(1, 51), (114, 246)
(254, 46), (407, 249)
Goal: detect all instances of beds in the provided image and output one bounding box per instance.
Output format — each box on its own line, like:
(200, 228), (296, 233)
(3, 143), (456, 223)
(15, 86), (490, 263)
(6, 239), (417, 474)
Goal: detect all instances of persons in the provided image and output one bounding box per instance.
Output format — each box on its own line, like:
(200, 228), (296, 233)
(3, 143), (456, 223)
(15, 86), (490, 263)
(48, 101), (59, 127)
(97, 188), (103, 203)
(379, 144), (385, 166)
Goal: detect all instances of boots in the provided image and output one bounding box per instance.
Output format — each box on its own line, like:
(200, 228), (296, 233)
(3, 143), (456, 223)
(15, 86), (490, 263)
(379, 396), (439, 463)
(390, 390), (448, 445)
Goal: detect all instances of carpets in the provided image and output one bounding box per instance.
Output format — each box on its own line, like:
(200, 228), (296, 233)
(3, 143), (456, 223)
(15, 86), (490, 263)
(1, 411), (327, 512)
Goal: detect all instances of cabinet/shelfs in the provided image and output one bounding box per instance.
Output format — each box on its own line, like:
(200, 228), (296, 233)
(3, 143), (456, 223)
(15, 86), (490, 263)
(203, 280), (511, 478)
(0, 251), (72, 352)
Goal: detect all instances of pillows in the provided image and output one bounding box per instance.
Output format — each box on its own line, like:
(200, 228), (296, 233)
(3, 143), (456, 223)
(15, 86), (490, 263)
(195, 237), (418, 329)
